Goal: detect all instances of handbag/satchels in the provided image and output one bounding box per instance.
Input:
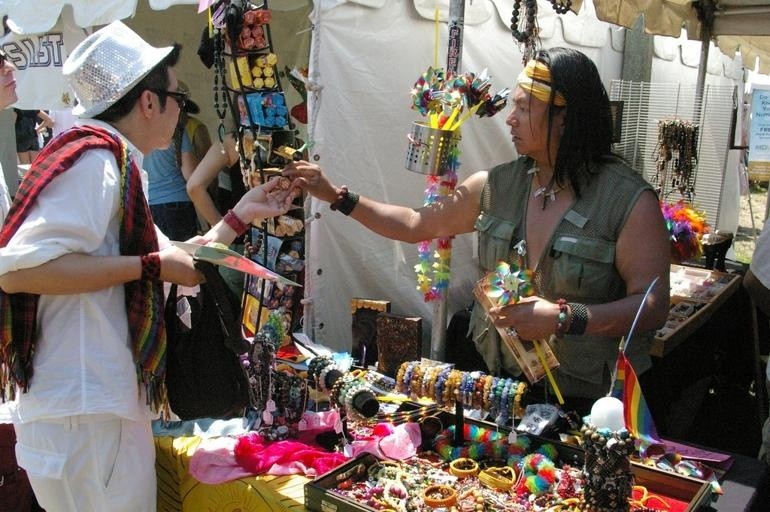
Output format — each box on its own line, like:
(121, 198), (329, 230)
(164, 262), (251, 421)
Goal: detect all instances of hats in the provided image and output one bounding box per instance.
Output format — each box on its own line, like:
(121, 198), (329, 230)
(60, 18), (175, 119)
(174, 79), (200, 114)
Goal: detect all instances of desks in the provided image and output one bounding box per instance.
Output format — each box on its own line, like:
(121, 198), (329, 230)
(136, 336), (760, 512)
(632, 261), (746, 441)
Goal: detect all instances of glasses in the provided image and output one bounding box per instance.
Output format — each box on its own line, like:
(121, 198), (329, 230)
(157, 90), (187, 110)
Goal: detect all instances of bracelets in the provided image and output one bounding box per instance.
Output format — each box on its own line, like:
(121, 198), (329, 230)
(330, 182), (348, 213)
(337, 188), (361, 217)
(553, 296), (568, 338)
(566, 301), (589, 338)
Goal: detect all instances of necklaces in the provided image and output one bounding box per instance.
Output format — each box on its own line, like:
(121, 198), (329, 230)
(525, 157), (591, 212)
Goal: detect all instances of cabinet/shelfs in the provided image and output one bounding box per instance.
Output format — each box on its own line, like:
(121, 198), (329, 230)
(209, 0), (308, 351)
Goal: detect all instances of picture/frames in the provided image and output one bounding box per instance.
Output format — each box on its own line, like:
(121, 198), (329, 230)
(603, 100), (625, 147)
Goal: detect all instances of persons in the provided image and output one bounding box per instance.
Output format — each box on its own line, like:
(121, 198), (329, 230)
(284, 44), (672, 426)
(1, 17), (304, 512)
(14, 77), (281, 344)
(741, 182), (769, 463)
(0, 46), (34, 512)
